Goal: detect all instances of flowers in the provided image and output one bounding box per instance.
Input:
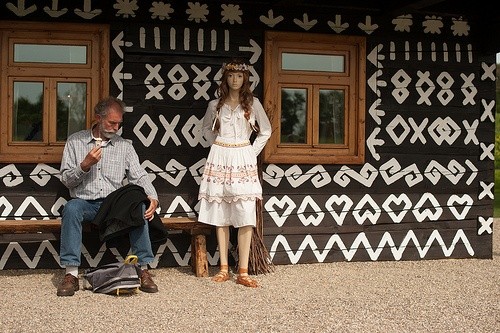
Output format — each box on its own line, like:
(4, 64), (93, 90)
(223, 62), (253, 71)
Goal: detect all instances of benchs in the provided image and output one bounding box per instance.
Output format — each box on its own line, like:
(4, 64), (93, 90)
(0, 216), (232, 278)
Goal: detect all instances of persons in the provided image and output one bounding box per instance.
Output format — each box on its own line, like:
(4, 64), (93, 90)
(57, 97), (160, 297)
(197, 60), (272, 287)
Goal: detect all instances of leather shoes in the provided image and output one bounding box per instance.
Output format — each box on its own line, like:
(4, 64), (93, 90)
(137, 269), (157, 293)
(57, 274), (78, 296)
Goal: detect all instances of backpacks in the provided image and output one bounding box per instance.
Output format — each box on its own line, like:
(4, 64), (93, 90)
(81, 255), (141, 296)
